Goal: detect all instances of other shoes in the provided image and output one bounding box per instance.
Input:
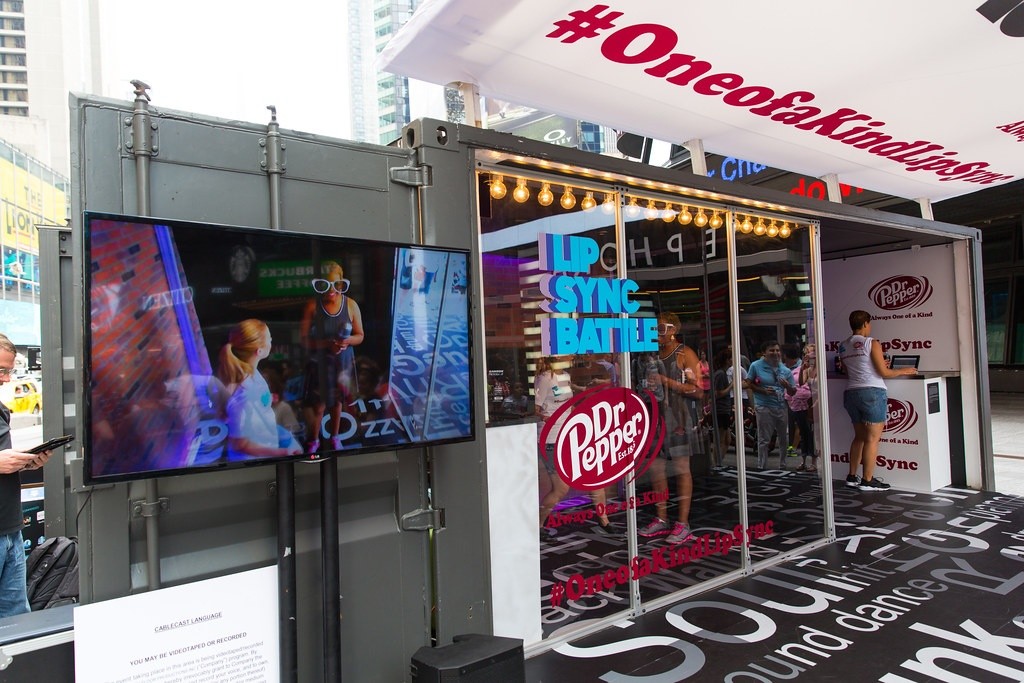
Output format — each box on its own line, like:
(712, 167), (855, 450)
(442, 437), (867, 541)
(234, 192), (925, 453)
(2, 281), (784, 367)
(845, 474), (862, 487)
(796, 464), (807, 470)
(309, 439), (320, 452)
(540, 533), (558, 544)
(330, 435), (344, 450)
(598, 522), (621, 534)
(806, 464), (817, 472)
(858, 476), (891, 490)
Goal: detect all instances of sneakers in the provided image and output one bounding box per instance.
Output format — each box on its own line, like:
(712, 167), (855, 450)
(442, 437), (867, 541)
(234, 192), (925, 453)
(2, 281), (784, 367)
(664, 520), (692, 544)
(638, 516), (672, 537)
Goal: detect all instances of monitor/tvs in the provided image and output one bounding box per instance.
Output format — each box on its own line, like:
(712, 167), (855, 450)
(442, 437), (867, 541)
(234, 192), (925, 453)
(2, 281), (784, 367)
(80, 207), (477, 486)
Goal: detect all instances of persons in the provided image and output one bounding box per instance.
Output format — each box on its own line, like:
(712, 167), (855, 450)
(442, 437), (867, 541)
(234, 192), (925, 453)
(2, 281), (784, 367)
(487, 367), (535, 426)
(572, 341), (821, 499)
(299, 261), (364, 453)
(397, 246), (468, 315)
(16, 386), (21, 393)
(535, 355), (621, 542)
(218, 319), (304, 463)
(839, 310), (919, 491)
(638, 313), (704, 544)
(0, 334), (54, 618)
(256, 354), (402, 437)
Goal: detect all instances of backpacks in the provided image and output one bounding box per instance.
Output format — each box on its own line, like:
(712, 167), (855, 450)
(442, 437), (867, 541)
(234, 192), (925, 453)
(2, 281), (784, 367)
(26, 536), (79, 612)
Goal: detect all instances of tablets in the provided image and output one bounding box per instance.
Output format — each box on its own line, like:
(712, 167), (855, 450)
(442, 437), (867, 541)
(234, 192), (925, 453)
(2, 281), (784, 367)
(26, 435), (75, 454)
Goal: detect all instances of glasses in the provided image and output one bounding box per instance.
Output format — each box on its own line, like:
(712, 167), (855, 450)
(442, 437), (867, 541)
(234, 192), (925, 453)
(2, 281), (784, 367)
(658, 323), (673, 335)
(0, 369), (17, 376)
(311, 279), (350, 293)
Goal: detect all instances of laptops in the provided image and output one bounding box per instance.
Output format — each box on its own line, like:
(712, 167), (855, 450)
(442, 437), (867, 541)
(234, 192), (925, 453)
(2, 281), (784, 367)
(890, 355), (920, 370)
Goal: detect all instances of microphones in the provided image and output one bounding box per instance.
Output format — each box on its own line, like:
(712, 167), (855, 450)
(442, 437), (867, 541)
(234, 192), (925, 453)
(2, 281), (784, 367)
(337, 320), (352, 341)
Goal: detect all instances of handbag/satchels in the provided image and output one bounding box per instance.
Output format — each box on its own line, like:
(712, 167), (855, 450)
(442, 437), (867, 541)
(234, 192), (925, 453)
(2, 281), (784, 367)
(835, 346), (842, 374)
